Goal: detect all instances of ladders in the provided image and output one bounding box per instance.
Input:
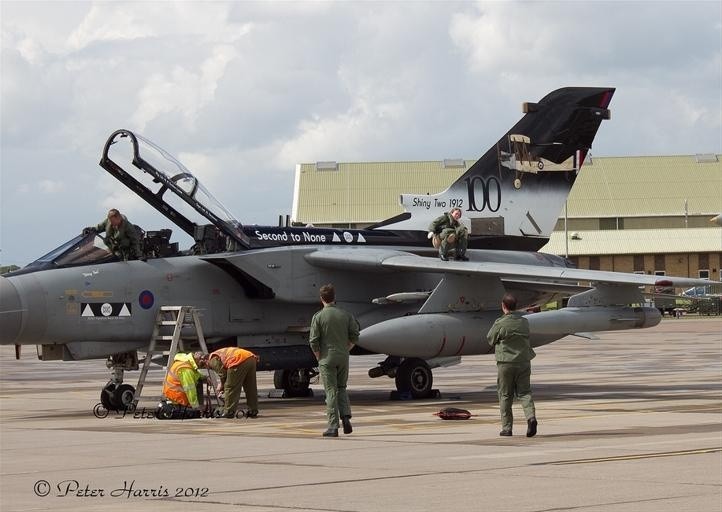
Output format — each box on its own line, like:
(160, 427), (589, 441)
(132, 305), (224, 410)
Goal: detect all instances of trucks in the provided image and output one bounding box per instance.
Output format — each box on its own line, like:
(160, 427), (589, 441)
(679, 286), (722, 297)
(652, 284), (676, 317)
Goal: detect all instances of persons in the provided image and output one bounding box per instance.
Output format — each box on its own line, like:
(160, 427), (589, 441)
(215, 220), (243, 252)
(199, 346), (258, 418)
(83, 209), (148, 262)
(487, 293), (537, 437)
(309, 283), (360, 437)
(427, 208), (470, 261)
(156, 350), (205, 419)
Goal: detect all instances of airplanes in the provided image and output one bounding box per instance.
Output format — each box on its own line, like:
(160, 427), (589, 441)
(495, 133), (584, 190)
(1, 86), (722, 411)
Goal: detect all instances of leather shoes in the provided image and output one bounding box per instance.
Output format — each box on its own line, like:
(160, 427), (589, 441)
(246, 410), (257, 418)
(453, 255), (470, 261)
(526, 417), (537, 437)
(441, 255), (449, 261)
(499, 430), (512, 436)
(342, 417), (352, 434)
(323, 429), (338, 436)
(216, 413), (234, 418)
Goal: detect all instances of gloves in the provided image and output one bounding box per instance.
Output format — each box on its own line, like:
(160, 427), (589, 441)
(427, 232), (436, 239)
